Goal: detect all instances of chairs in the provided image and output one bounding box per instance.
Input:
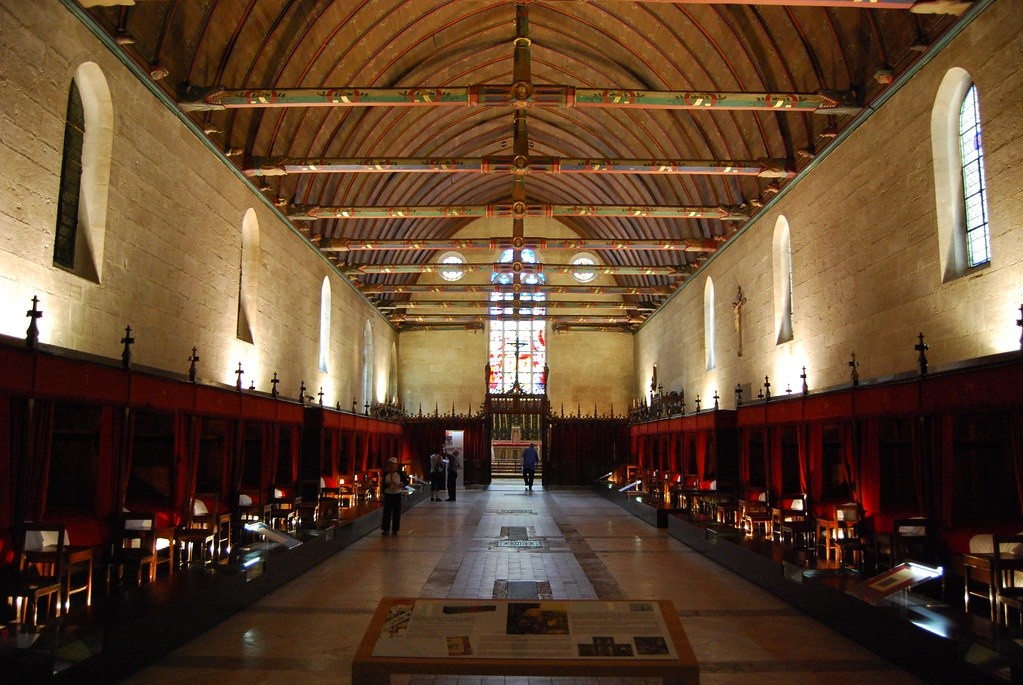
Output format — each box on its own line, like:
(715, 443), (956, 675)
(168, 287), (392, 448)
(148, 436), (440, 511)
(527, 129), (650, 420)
(613, 463), (1023, 632)
(0, 468), (384, 639)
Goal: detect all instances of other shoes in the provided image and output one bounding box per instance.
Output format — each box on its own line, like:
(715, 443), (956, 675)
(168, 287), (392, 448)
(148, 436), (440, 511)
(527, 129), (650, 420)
(381, 531), (389, 536)
(529, 486), (532, 490)
(436, 498), (443, 502)
(392, 531), (398, 535)
(525, 482), (529, 486)
(431, 497), (434, 501)
(445, 498), (456, 501)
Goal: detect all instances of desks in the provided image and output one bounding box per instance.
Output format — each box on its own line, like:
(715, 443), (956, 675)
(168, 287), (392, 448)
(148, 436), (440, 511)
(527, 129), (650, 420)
(192, 512), (233, 561)
(738, 499), (767, 533)
(961, 551), (1023, 623)
(770, 507), (805, 542)
(663, 479), (677, 503)
(25, 545), (95, 615)
(123, 524), (177, 582)
(814, 517), (859, 567)
(239, 505), (269, 543)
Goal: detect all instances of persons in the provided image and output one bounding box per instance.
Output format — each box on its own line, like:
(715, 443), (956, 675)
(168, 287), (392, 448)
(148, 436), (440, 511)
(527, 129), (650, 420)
(522, 443), (539, 486)
(544, 362), (549, 381)
(731, 302), (742, 333)
(381, 457), (408, 536)
(431, 448), (460, 501)
(485, 362), (491, 382)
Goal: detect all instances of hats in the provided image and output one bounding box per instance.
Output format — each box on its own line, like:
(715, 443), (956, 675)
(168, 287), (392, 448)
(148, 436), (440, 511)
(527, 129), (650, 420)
(388, 457), (397, 463)
